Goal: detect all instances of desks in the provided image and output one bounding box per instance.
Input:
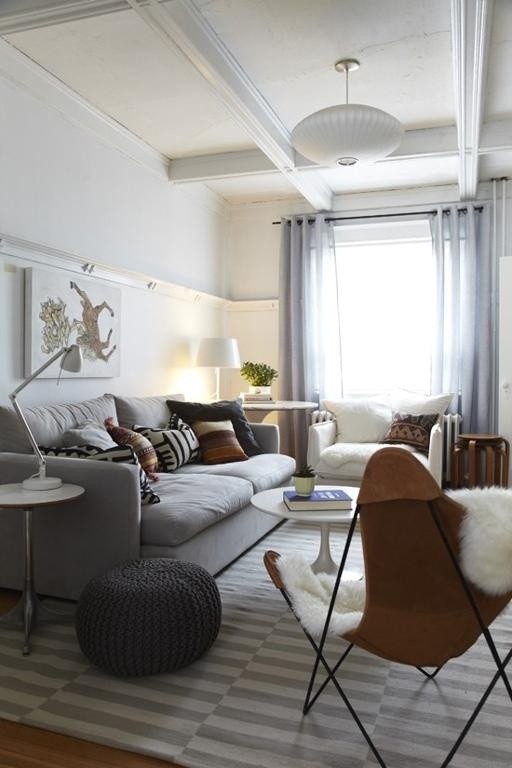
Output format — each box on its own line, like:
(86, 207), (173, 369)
(241, 400), (319, 423)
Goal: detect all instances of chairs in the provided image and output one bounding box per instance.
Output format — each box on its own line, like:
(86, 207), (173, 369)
(263, 447), (512, 768)
(306, 418), (444, 489)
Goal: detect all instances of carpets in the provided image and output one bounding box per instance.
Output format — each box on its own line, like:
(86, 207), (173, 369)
(0, 518), (511, 768)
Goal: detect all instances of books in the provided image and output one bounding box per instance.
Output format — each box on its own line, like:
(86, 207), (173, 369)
(241, 400), (276, 404)
(241, 393), (273, 401)
(283, 489), (353, 509)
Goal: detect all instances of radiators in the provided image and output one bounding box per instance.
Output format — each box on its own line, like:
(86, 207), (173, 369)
(311, 411), (463, 482)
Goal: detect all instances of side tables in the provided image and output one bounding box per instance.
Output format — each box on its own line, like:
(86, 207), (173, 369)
(0, 482), (86, 656)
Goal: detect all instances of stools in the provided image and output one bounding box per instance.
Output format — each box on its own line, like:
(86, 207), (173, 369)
(75, 558), (221, 677)
(452, 435), (510, 489)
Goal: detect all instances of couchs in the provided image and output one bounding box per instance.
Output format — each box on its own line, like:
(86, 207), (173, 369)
(0, 423), (292, 601)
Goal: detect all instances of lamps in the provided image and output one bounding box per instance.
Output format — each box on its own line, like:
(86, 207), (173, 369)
(196, 338), (241, 401)
(8, 345), (82, 490)
(290, 59), (404, 166)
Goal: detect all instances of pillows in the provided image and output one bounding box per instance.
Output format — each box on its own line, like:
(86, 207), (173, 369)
(0, 393), (264, 506)
(321, 393), (454, 453)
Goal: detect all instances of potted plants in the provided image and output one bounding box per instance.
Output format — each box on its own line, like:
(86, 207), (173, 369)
(241, 362), (279, 395)
(292, 464), (317, 497)
